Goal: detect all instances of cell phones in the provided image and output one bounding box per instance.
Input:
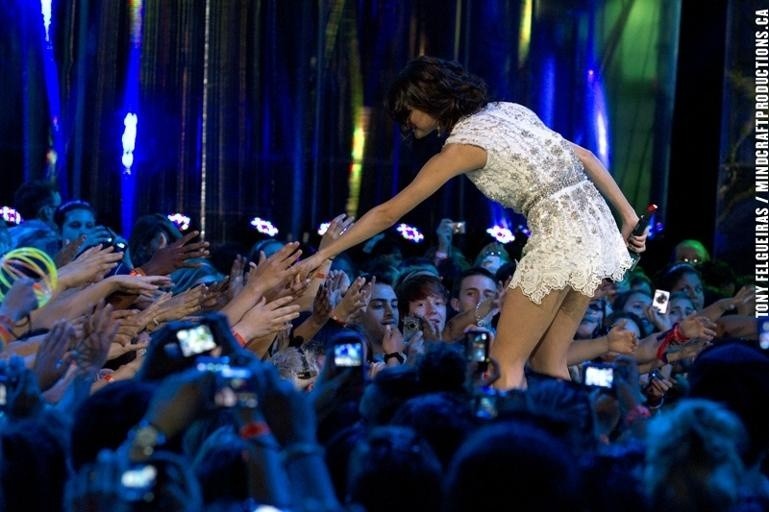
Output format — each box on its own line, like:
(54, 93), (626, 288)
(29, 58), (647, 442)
(446, 219), (466, 236)
(100, 226), (128, 253)
(652, 287), (671, 313)
(403, 316), (422, 340)
(650, 366), (665, 387)
(175, 319), (234, 366)
(583, 364), (613, 388)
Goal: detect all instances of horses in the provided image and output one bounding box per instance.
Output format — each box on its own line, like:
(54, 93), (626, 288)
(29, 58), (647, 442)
(464, 328), (488, 366)
(331, 337), (367, 369)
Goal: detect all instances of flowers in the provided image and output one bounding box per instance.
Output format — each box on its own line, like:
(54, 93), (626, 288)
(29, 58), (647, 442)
(626, 201), (660, 272)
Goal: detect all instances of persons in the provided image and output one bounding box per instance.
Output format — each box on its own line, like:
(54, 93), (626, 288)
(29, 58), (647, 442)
(0, 159), (768, 510)
(295, 57), (647, 395)
